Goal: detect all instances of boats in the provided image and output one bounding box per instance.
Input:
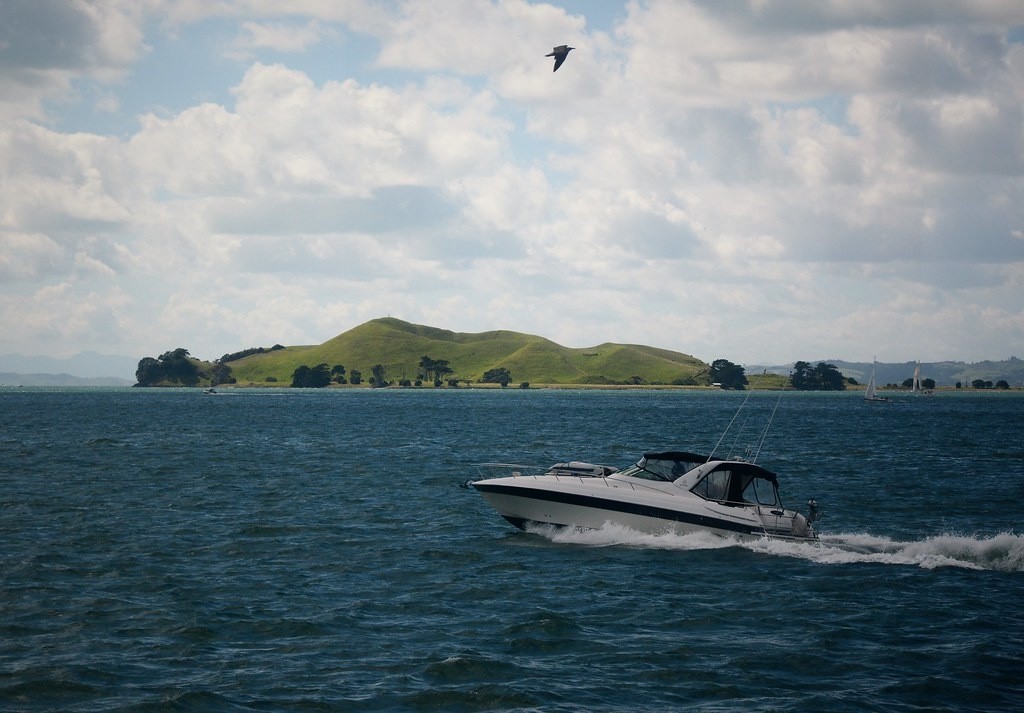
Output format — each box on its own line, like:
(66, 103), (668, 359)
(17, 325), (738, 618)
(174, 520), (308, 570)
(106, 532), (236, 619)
(469, 365), (830, 548)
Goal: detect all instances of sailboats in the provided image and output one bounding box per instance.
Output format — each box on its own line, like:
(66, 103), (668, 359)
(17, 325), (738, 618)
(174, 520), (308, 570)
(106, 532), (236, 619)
(863, 355), (888, 401)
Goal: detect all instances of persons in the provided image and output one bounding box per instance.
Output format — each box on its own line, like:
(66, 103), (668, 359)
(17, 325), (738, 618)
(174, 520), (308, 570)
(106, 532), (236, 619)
(671, 461), (687, 480)
